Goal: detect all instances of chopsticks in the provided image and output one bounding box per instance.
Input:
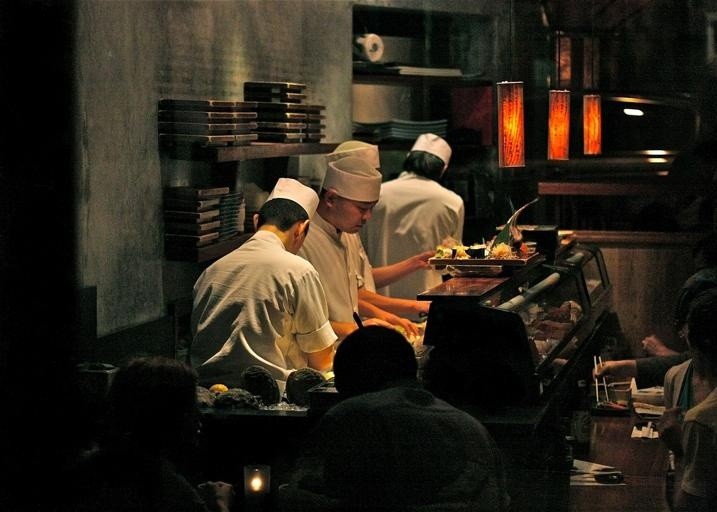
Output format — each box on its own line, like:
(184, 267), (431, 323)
(593, 354), (611, 404)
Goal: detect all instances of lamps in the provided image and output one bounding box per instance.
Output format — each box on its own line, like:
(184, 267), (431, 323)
(493, 1), (602, 169)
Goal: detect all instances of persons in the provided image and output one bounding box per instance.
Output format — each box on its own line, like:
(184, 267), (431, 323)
(325, 137), (443, 314)
(188, 176), (336, 380)
(591, 234), (717, 511)
(291, 155), (420, 343)
(358, 132), (466, 321)
(51, 355), (234, 510)
(273, 324), (513, 511)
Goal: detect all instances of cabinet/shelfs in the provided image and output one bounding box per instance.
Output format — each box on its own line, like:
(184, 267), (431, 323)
(350, 5), (494, 184)
(419, 241), (611, 405)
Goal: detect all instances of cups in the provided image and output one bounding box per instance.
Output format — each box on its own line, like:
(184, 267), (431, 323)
(242, 464), (271, 496)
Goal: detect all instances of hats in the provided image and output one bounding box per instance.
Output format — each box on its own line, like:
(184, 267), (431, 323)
(411, 133), (452, 165)
(323, 140), (382, 202)
(265, 178), (319, 219)
